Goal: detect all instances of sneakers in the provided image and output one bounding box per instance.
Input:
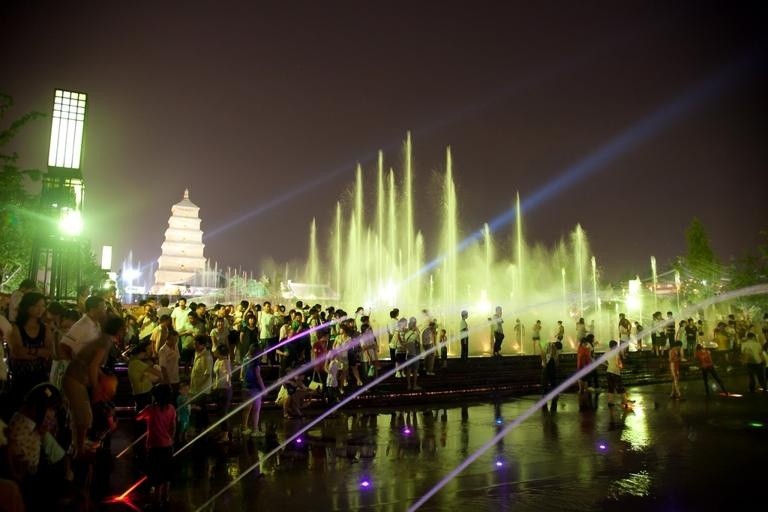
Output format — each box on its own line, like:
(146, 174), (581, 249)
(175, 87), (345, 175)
(396, 365), (447, 390)
(241, 428), (266, 437)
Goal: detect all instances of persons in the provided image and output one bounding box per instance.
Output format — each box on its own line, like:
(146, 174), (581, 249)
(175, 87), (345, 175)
(541, 333), (629, 417)
(514, 318), (591, 355)
(0, 287), (380, 512)
(460, 310), (469, 361)
(619, 312), (768, 401)
(493, 306), (504, 360)
(386, 308), (448, 390)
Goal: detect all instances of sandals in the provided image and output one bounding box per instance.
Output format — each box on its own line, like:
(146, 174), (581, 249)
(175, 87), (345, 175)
(283, 411), (303, 419)
(215, 436), (231, 444)
